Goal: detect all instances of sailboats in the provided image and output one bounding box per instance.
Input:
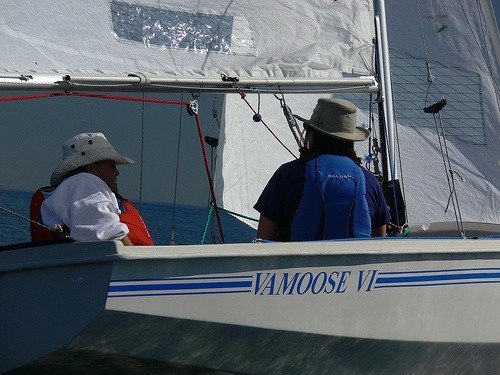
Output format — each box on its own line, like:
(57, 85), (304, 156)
(0, 0), (500, 375)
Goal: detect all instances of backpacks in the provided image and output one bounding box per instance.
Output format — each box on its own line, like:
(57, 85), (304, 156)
(289, 154), (371, 242)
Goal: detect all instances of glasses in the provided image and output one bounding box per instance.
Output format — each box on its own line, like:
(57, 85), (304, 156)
(303, 122), (315, 132)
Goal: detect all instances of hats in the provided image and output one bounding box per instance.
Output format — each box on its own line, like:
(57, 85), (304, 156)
(292, 98), (371, 141)
(50, 132), (136, 186)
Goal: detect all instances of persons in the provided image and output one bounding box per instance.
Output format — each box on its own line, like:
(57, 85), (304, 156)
(41, 133), (134, 246)
(253, 98), (392, 240)
